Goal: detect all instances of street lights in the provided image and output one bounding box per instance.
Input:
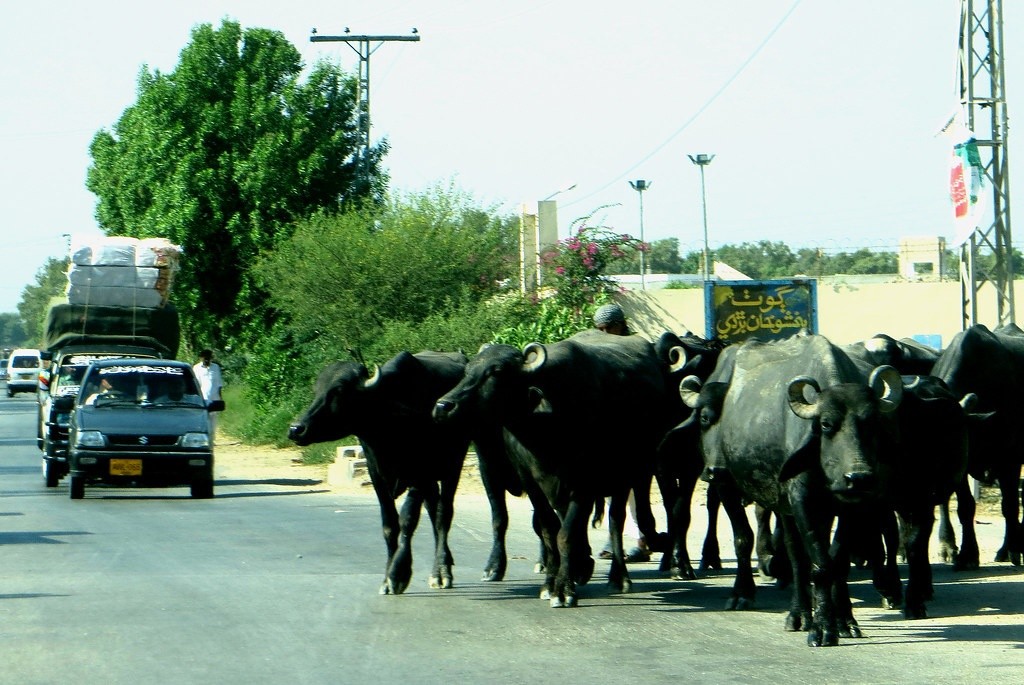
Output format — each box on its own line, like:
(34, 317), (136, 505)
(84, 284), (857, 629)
(687, 153), (716, 282)
(629, 179), (651, 290)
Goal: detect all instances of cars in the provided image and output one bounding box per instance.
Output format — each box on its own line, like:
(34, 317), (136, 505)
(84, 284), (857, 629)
(1, 359), (9, 378)
(53, 358), (226, 499)
(34, 344), (165, 489)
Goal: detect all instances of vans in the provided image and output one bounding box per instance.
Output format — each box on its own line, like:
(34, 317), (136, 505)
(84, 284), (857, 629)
(5, 348), (45, 397)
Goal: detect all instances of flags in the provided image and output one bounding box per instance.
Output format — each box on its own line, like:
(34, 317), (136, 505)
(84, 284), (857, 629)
(915, 120), (986, 250)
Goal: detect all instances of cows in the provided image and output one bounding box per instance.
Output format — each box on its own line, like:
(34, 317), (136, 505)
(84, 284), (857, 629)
(284, 320), (1024, 648)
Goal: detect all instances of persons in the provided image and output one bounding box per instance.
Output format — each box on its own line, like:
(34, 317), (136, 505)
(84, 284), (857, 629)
(85, 375), (122, 405)
(192, 349), (224, 449)
(58, 366), (84, 386)
(593, 305), (655, 563)
(153, 375), (203, 407)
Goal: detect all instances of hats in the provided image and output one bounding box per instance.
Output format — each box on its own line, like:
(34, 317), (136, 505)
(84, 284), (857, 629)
(593, 304), (624, 323)
